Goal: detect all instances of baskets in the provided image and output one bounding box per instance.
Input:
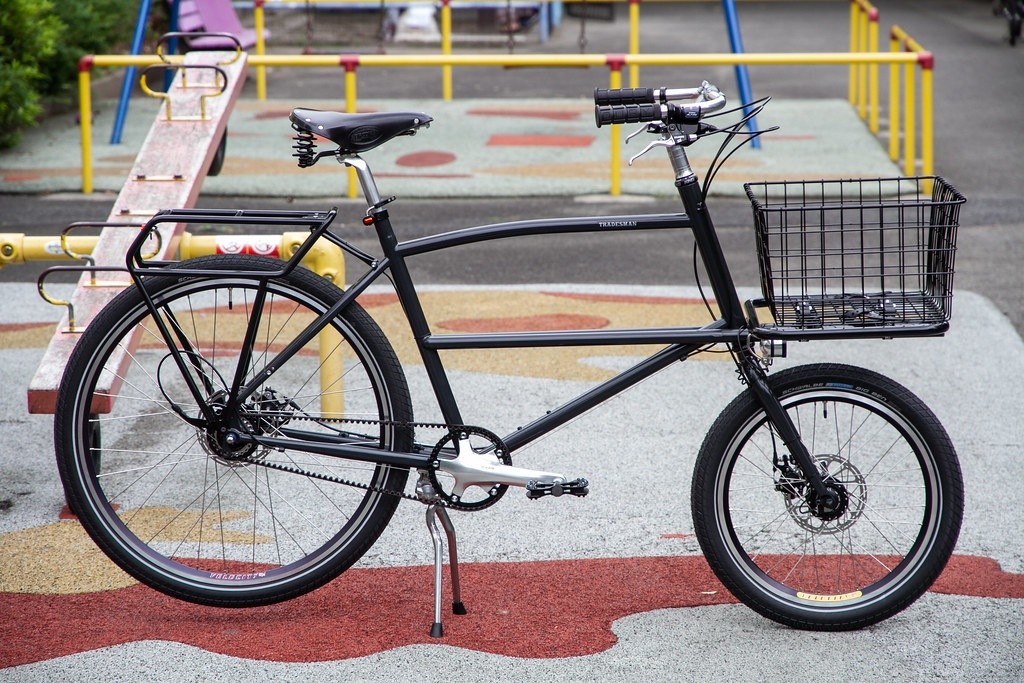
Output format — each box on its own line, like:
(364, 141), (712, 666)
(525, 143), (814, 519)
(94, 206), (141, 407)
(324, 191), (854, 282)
(744, 175), (967, 337)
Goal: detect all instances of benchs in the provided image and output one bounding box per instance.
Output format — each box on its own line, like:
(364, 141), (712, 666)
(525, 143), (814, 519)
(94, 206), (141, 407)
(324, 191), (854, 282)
(169, 0), (271, 50)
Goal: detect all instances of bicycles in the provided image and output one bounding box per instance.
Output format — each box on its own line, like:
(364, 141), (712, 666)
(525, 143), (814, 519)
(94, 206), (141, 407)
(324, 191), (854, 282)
(48, 79), (967, 640)
(992, 0), (1024, 48)
